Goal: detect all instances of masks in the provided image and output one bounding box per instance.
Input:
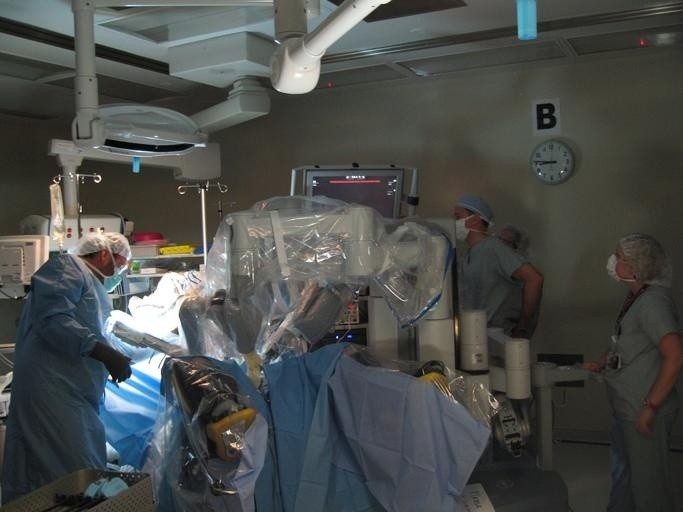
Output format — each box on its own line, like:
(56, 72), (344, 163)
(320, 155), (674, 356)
(455, 214), (475, 243)
(606, 254), (636, 283)
(100, 260), (122, 292)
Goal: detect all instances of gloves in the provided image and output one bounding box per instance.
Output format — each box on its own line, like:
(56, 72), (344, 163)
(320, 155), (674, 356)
(90, 341), (132, 382)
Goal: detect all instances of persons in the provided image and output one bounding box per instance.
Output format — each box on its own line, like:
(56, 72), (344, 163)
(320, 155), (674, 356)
(583, 229), (682, 512)
(454, 193), (545, 340)
(5, 230), (134, 503)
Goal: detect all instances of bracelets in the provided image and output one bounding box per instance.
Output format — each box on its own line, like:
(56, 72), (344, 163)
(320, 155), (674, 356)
(642, 397), (660, 412)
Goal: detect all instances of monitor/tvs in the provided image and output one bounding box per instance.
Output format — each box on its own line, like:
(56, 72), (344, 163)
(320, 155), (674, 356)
(304, 169), (403, 218)
(0, 235), (49, 299)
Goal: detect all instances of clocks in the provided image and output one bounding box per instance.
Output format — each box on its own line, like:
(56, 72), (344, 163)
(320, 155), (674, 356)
(529, 139), (576, 187)
